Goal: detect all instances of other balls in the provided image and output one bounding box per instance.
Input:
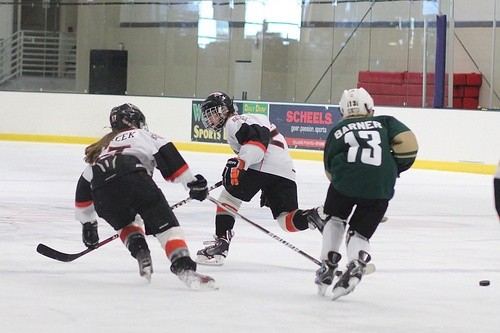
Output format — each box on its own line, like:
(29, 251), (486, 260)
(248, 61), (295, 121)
(479, 280), (491, 287)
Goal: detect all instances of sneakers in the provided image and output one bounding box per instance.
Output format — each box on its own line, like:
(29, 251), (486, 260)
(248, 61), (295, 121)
(129, 233), (153, 282)
(195, 229), (235, 265)
(315, 251), (342, 295)
(170, 257), (219, 291)
(331, 250), (372, 300)
(302, 201), (329, 235)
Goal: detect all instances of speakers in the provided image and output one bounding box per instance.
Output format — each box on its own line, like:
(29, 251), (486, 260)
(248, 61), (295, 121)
(89, 49), (128, 96)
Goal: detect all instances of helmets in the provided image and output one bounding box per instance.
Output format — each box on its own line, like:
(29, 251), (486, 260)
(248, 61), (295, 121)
(110, 102), (146, 131)
(338, 88), (373, 117)
(200, 92), (236, 133)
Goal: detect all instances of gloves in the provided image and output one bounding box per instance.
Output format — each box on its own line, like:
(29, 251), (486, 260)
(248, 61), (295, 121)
(187, 173), (209, 202)
(81, 220), (99, 250)
(221, 158), (247, 191)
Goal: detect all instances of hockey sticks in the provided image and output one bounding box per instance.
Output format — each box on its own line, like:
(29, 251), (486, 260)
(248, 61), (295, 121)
(206, 194), (377, 278)
(36, 179), (223, 263)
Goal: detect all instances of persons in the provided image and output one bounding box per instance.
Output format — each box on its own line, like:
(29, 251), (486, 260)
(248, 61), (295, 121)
(196, 90), (331, 265)
(74, 102), (215, 292)
(314, 86), (419, 300)
